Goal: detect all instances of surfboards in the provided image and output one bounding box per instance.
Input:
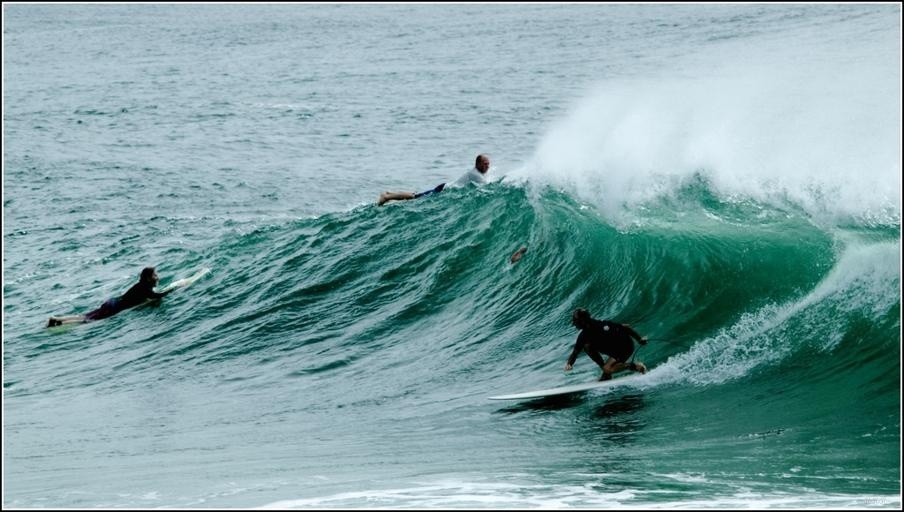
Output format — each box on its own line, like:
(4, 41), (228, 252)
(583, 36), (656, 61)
(487, 378), (626, 400)
(50, 268), (211, 336)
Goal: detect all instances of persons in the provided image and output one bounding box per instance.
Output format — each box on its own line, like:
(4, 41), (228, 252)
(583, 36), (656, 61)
(565, 308), (648, 382)
(379, 154), (490, 207)
(48, 265), (173, 329)
